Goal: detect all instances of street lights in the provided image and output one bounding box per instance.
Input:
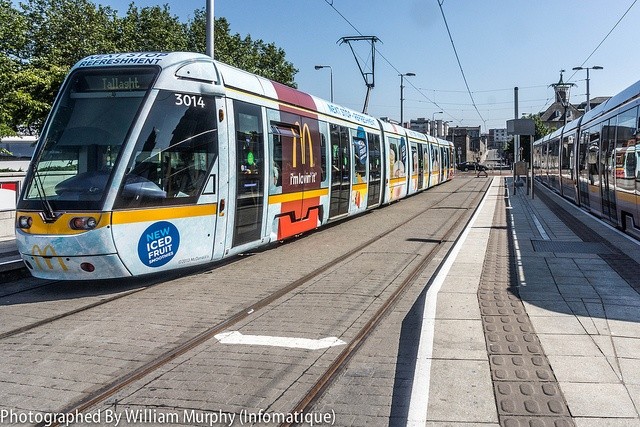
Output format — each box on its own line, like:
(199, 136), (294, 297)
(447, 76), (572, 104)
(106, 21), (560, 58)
(399, 72), (416, 126)
(572, 66), (604, 113)
(315, 65), (333, 102)
(433, 111), (443, 137)
(443, 120), (453, 139)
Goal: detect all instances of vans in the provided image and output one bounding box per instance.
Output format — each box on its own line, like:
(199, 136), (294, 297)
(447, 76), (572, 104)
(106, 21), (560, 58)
(0, 134), (80, 212)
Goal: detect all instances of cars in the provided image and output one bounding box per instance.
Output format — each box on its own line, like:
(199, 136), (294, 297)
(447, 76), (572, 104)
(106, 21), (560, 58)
(457, 161), (488, 172)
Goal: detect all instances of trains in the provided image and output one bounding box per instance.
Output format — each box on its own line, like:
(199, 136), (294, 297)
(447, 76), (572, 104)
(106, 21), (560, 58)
(15, 51), (456, 280)
(532, 81), (640, 262)
